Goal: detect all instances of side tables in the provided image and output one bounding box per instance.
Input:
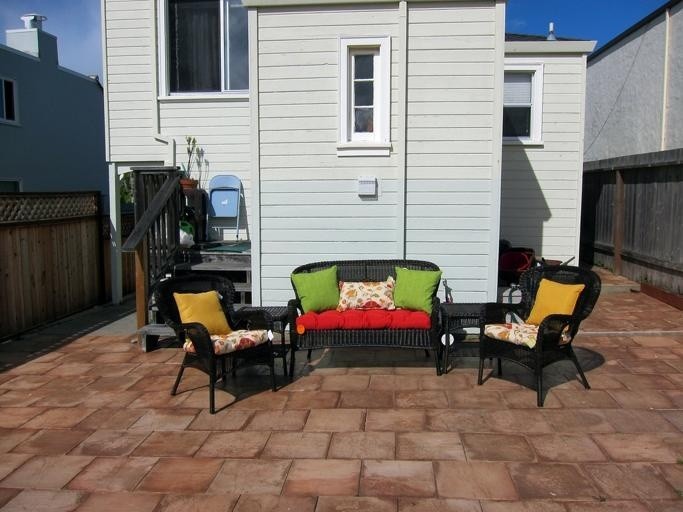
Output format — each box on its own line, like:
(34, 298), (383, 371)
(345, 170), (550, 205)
(240, 307), (290, 384)
(440, 302), (484, 375)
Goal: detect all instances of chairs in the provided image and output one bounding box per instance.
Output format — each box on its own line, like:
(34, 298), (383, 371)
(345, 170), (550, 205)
(478, 266), (601, 408)
(206, 175), (242, 243)
(155, 274), (276, 415)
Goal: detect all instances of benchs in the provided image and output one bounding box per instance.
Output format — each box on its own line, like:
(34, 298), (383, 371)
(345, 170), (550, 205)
(288, 260), (442, 379)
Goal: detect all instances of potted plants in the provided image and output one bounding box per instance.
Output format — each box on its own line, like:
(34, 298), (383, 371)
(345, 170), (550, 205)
(181, 136), (200, 190)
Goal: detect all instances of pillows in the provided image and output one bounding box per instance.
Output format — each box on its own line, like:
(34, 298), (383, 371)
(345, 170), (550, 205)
(291, 265), (340, 313)
(337, 276), (395, 310)
(174, 290), (232, 337)
(394, 267), (442, 314)
(525, 278), (585, 329)
